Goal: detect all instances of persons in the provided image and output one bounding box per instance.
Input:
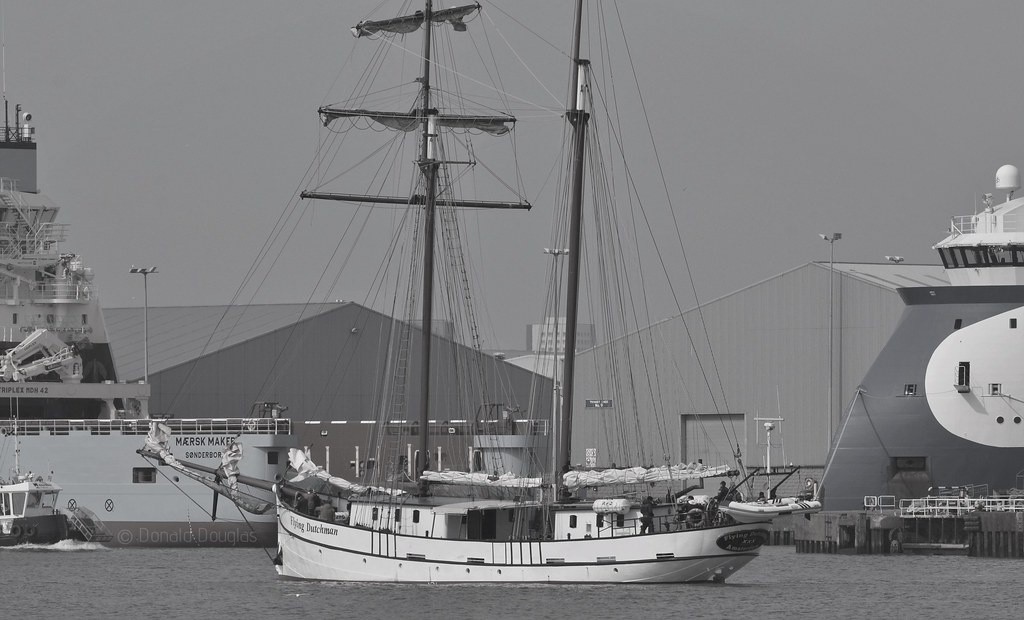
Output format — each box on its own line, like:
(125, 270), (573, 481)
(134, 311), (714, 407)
(639, 495), (656, 536)
(674, 477), (766, 530)
(292, 487), (337, 525)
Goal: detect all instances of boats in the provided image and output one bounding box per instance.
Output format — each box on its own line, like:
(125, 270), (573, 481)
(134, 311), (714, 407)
(715, 493), (822, 523)
(791, 163), (1024, 560)
(1, 79), (295, 542)
(0, 406), (84, 548)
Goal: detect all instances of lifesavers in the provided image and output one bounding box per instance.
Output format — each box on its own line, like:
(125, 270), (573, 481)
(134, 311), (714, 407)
(685, 507), (706, 529)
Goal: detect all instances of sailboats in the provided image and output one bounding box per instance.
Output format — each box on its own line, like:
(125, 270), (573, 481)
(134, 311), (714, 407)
(137, 0), (775, 584)
